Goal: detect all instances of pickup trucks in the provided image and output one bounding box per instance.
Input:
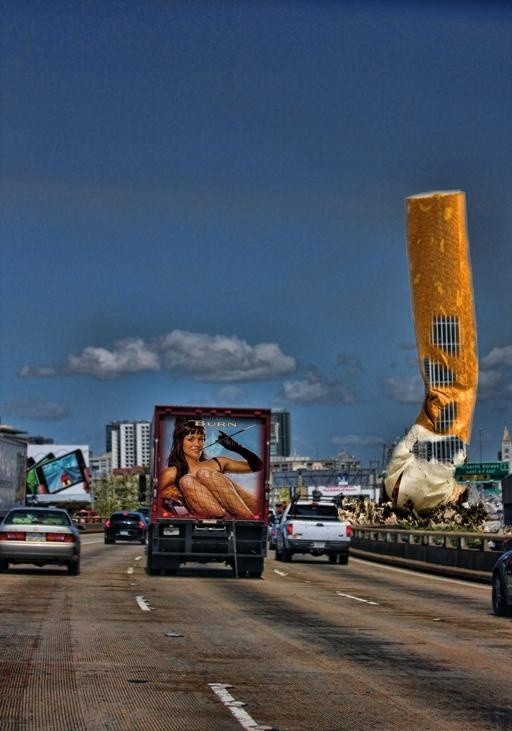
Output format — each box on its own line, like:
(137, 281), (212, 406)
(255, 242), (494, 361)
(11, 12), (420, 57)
(276, 497), (352, 563)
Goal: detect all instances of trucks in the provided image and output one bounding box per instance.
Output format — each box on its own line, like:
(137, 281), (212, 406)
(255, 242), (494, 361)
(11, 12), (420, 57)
(145, 405), (270, 575)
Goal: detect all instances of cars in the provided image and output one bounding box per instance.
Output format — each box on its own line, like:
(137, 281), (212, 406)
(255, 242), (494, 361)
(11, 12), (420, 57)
(103, 511), (148, 544)
(0, 508), (80, 574)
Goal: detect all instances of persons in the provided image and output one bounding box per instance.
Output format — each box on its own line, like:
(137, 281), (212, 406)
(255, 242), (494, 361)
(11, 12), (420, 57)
(159, 415), (263, 521)
